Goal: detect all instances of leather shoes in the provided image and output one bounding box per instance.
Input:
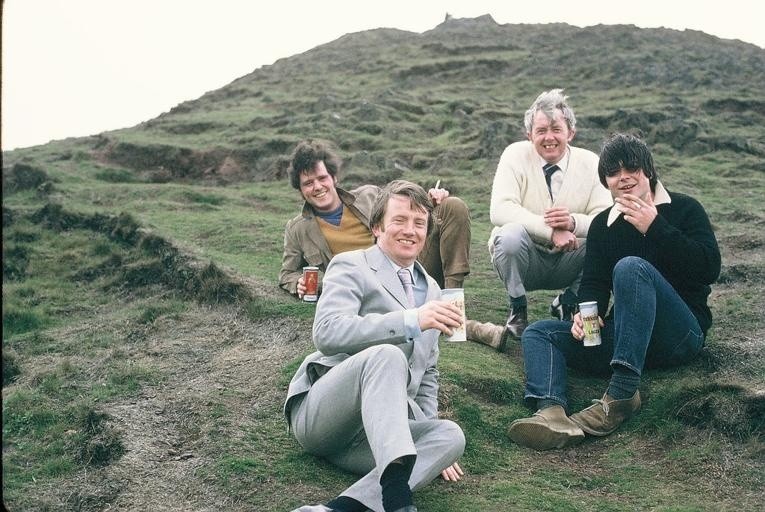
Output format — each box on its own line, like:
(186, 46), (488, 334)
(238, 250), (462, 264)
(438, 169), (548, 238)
(506, 302), (528, 340)
(549, 294), (575, 321)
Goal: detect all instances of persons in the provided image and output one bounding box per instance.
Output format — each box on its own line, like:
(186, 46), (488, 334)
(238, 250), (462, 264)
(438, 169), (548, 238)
(284, 180), (466, 512)
(508, 134), (721, 451)
(488, 88), (614, 340)
(279, 140), (509, 351)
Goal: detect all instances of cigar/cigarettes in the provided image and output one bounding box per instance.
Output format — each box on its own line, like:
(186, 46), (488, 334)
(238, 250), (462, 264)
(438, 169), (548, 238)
(631, 201), (642, 210)
(435, 180), (441, 190)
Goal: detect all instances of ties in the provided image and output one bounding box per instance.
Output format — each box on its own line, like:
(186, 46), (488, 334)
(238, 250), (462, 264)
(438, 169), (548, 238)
(398, 270), (415, 309)
(543, 164), (558, 200)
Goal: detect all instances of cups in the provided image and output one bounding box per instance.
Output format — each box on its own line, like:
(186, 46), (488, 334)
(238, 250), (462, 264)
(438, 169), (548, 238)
(302, 266), (318, 303)
(438, 288), (468, 344)
(577, 300), (605, 347)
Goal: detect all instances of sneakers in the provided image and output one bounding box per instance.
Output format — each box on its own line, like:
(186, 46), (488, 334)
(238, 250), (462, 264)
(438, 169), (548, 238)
(466, 320), (508, 350)
(507, 386), (641, 450)
